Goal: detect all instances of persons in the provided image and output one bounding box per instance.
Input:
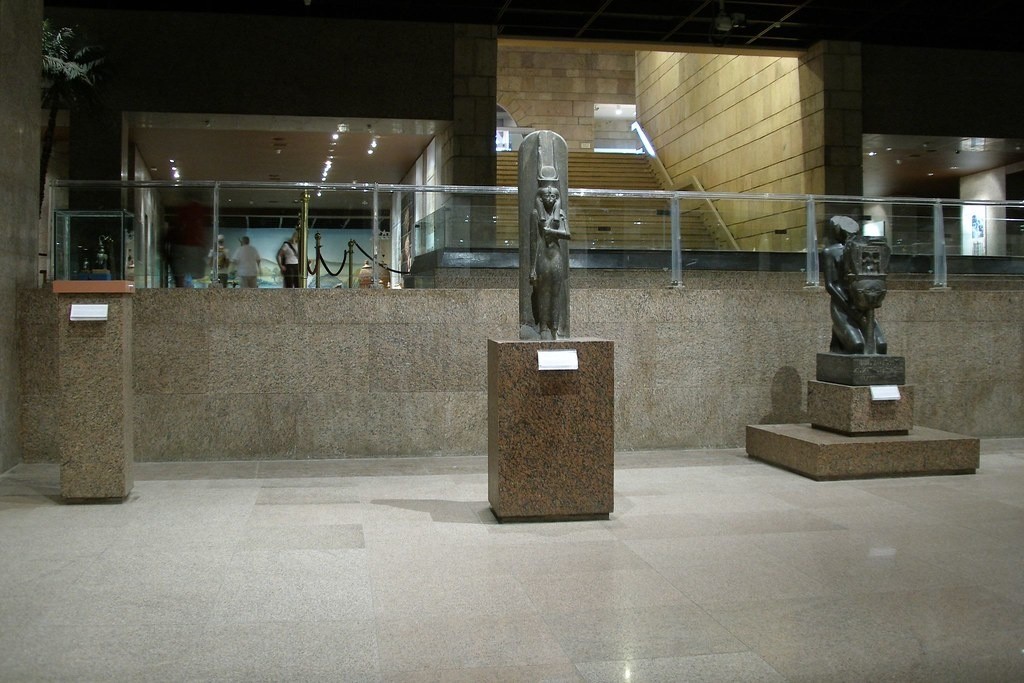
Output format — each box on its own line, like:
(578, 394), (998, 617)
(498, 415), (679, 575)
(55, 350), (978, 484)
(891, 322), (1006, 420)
(528, 187), (572, 340)
(823, 215), (887, 354)
(208, 234), (390, 289)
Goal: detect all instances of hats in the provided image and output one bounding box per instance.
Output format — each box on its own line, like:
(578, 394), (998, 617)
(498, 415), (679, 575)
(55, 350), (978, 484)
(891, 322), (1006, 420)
(217, 234), (224, 242)
